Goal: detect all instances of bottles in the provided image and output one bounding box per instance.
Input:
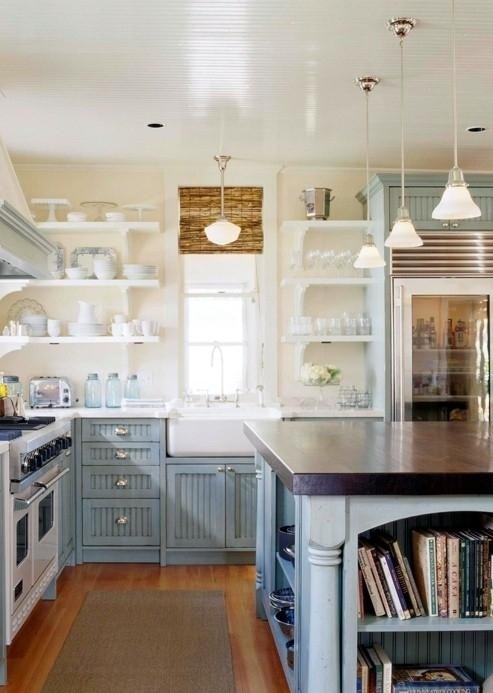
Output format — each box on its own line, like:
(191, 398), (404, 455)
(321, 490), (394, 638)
(413, 377), (474, 396)
(105, 371), (122, 408)
(123, 373), (139, 398)
(411, 312), (480, 347)
(1, 375), (20, 395)
(82, 372), (102, 408)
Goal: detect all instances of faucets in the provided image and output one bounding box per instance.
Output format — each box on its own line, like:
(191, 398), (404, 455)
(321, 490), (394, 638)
(209, 344), (228, 402)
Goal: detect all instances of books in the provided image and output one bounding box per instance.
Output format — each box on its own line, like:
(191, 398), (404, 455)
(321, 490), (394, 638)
(357, 526), (492, 692)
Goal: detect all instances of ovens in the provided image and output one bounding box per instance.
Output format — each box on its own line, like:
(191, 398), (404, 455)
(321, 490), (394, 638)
(7, 447), (71, 641)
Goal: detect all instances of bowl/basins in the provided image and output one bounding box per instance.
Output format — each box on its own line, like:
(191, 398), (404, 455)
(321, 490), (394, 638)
(93, 257), (116, 278)
(265, 586), (296, 608)
(283, 638), (294, 669)
(272, 610), (294, 637)
(65, 211), (86, 221)
(64, 266), (88, 279)
(104, 211), (126, 222)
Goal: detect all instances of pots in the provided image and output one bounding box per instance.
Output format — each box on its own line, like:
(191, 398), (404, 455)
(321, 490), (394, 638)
(277, 525), (294, 568)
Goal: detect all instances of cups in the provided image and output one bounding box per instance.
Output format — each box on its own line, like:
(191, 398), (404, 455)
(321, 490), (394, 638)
(338, 384), (369, 408)
(51, 270), (64, 279)
(285, 315), (371, 336)
(106, 314), (159, 336)
(46, 318), (62, 337)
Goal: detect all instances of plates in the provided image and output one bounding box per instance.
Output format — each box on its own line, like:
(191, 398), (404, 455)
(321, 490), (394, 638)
(121, 263), (159, 280)
(66, 321), (106, 337)
(23, 312), (47, 337)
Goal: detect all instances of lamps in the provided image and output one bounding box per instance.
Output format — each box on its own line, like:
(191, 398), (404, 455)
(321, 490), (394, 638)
(352, 72), (386, 268)
(382, 13), (424, 248)
(201, 155), (242, 248)
(430, 0), (480, 225)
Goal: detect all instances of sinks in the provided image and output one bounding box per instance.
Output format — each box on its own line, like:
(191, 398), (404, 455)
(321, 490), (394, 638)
(164, 400), (281, 458)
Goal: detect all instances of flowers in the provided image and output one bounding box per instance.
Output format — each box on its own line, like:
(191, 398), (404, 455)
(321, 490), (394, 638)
(298, 361), (341, 387)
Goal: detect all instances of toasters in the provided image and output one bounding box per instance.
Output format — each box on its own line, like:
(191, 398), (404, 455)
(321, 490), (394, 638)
(28, 375), (73, 408)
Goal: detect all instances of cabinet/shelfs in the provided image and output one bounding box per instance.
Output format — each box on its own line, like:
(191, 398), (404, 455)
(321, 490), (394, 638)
(278, 218), (375, 372)
(355, 170), (492, 420)
(44, 416), (77, 598)
(160, 455), (262, 565)
(77, 418), (166, 565)
(0, 218), (164, 363)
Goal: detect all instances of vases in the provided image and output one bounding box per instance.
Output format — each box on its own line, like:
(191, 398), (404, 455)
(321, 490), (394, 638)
(313, 389), (329, 411)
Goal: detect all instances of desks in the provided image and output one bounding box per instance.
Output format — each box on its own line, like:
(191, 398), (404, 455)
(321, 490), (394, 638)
(239, 417), (491, 692)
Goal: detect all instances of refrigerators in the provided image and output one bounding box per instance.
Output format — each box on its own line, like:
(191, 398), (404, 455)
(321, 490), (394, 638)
(389, 276), (491, 420)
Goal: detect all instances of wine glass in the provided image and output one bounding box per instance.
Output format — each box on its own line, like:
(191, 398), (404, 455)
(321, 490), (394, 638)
(304, 247), (368, 278)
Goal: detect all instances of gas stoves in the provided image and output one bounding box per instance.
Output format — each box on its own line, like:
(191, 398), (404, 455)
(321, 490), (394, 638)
(0, 415), (72, 483)
